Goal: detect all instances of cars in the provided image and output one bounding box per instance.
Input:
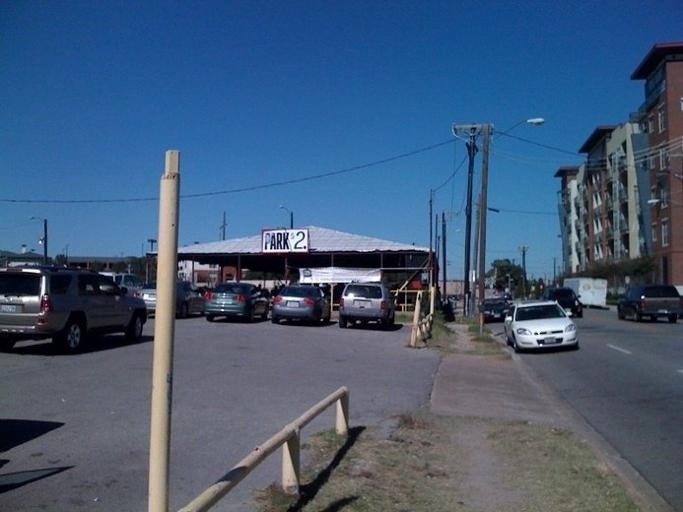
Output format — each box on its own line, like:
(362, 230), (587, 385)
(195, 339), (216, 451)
(483, 284), (588, 356)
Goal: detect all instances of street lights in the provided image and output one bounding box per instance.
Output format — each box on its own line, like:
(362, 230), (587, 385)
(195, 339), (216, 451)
(476, 112), (546, 336)
(279, 204), (294, 229)
(27, 215), (48, 263)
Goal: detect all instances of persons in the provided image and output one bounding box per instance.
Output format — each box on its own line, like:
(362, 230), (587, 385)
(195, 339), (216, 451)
(258, 284), (285, 309)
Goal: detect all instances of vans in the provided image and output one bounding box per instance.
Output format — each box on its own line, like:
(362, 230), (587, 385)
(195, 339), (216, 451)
(616, 282), (683, 324)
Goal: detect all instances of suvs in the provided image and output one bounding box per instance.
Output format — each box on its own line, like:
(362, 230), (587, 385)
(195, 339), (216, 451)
(0, 260), (149, 356)
